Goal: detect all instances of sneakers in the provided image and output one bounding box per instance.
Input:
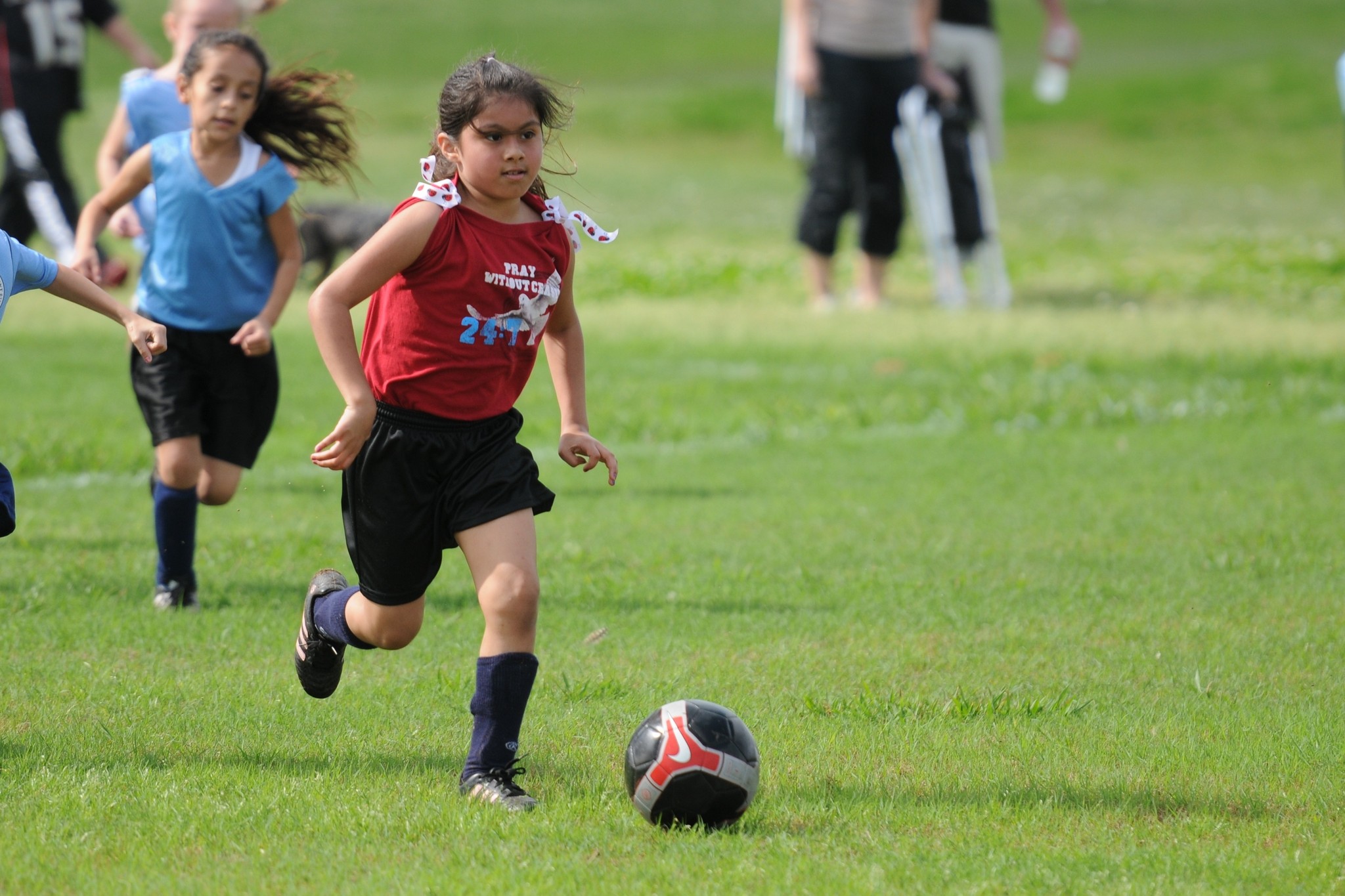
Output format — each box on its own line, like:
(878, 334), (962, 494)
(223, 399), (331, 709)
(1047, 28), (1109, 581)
(157, 584), (200, 617)
(295, 569), (349, 700)
(457, 769), (540, 814)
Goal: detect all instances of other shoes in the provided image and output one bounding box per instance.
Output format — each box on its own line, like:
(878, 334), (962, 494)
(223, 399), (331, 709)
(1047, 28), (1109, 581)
(810, 290), (882, 317)
(93, 255), (127, 289)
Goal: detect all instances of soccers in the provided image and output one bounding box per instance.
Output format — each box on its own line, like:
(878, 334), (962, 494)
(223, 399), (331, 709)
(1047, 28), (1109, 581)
(623, 697), (763, 832)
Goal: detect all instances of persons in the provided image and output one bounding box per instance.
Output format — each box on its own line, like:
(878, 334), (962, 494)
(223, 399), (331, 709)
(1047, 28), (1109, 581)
(0, 226), (171, 541)
(775, 0), (934, 312)
(294, 48), (621, 816)
(1, 0), (162, 291)
(916, 1), (1083, 273)
(94, 1), (279, 255)
(70, 28), (366, 616)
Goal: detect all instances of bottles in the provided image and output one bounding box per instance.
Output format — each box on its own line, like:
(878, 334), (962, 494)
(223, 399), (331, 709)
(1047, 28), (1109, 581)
(1037, 26), (1076, 101)
